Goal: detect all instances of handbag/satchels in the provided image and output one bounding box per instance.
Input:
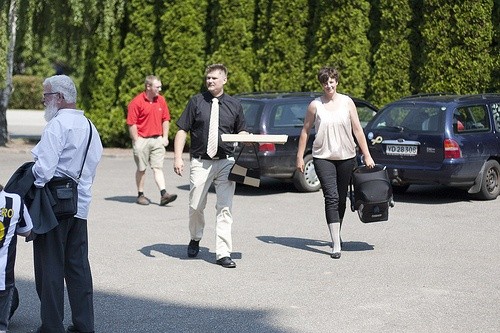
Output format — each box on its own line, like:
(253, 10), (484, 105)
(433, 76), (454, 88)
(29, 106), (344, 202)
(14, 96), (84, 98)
(228, 143), (260, 187)
(49, 180), (78, 216)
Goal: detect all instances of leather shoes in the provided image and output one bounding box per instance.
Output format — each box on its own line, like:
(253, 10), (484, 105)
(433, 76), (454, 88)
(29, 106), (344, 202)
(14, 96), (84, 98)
(216, 257), (236, 267)
(188, 240), (199, 258)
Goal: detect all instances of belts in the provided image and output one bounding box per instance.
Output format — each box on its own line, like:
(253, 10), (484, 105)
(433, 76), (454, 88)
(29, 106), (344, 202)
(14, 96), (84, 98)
(143, 135), (159, 139)
(193, 154), (235, 160)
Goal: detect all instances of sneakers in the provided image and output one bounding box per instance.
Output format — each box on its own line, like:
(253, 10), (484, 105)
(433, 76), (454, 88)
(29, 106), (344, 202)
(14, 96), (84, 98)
(161, 193), (177, 205)
(137, 195), (149, 205)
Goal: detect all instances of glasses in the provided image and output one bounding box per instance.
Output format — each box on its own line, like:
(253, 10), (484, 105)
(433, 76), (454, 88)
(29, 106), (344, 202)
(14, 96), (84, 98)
(42, 92), (65, 99)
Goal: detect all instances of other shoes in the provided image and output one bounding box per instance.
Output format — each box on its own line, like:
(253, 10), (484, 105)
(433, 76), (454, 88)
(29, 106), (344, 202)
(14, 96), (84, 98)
(331, 242), (342, 248)
(331, 253), (341, 259)
(68, 325), (73, 332)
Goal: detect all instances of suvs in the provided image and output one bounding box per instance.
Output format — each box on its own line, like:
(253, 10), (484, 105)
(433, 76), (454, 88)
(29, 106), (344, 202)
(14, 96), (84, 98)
(233, 91), (381, 193)
(357, 93), (500, 201)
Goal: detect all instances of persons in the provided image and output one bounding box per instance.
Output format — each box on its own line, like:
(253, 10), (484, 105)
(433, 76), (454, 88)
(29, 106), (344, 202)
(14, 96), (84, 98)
(296, 67), (376, 259)
(0, 184), (35, 332)
(174, 65), (254, 268)
(127, 76), (177, 206)
(31, 75), (104, 333)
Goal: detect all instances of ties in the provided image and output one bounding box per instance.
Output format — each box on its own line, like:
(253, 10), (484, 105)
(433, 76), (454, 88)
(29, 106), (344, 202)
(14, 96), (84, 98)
(207, 98), (221, 158)
